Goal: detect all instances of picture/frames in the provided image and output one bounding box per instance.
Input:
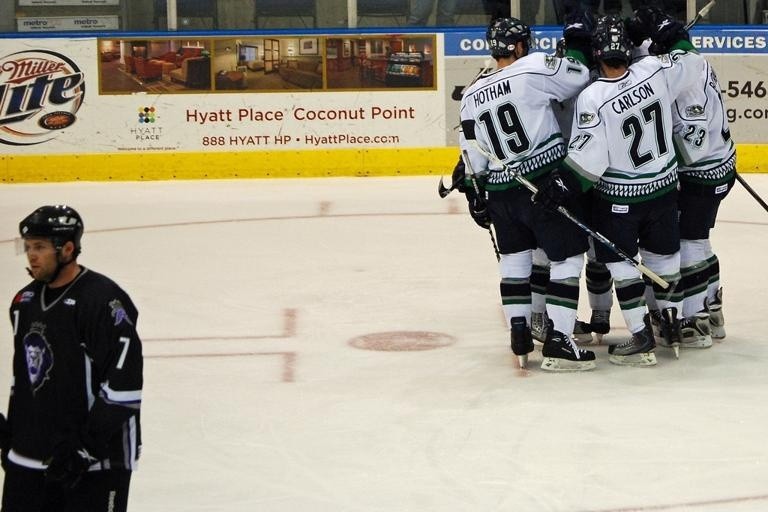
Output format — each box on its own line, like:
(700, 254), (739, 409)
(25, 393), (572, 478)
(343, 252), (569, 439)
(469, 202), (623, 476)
(344, 43), (350, 55)
(299, 38), (318, 55)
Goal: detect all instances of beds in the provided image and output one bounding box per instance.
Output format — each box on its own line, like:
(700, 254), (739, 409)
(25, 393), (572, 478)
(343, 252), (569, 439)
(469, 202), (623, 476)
(247, 54), (264, 72)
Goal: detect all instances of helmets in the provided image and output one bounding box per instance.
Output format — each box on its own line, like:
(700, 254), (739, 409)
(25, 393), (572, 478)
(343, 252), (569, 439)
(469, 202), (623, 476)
(562, 8), (598, 42)
(486, 16), (531, 58)
(19, 204), (83, 240)
(591, 14), (635, 61)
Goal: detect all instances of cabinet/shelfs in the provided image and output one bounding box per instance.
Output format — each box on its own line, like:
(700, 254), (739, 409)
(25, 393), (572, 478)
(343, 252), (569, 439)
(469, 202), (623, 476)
(385, 50), (425, 87)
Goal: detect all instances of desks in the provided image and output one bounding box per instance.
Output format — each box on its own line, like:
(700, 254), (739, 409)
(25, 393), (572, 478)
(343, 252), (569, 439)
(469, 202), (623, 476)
(216, 72), (244, 90)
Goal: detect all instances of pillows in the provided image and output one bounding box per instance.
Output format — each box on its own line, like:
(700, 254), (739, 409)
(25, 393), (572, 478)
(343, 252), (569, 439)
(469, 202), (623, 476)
(316, 64), (322, 74)
(288, 60), (298, 69)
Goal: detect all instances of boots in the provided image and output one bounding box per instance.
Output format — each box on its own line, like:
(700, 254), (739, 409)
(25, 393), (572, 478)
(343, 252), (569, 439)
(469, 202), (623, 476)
(662, 307), (682, 347)
(608, 313), (656, 356)
(510, 316), (534, 355)
(542, 319), (595, 361)
(708, 287), (726, 339)
(679, 296), (713, 348)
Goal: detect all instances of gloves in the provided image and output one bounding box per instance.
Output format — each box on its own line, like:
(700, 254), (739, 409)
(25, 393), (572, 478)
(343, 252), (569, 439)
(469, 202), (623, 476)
(44, 447), (90, 490)
(469, 197), (496, 229)
(452, 154), (467, 193)
(534, 174), (574, 217)
(631, 0), (689, 56)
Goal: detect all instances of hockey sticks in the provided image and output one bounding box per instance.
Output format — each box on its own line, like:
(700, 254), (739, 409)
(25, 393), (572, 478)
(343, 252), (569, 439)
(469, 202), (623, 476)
(461, 119), (669, 289)
(452, 86), (465, 100)
(438, 175), (464, 198)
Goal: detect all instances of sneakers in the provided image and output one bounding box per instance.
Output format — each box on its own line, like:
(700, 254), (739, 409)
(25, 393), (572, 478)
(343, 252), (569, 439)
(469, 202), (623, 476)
(530, 310), (554, 343)
(572, 319), (593, 334)
(649, 308), (665, 338)
(590, 309), (611, 334)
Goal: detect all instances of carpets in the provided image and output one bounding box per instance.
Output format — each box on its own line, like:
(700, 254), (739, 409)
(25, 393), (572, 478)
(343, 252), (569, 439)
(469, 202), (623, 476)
(117, 66), (187, 91)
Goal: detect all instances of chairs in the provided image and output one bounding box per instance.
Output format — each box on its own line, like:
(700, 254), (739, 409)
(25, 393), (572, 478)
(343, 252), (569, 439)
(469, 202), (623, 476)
(236, 65), (248, 73)
(133, 52), (210, 90)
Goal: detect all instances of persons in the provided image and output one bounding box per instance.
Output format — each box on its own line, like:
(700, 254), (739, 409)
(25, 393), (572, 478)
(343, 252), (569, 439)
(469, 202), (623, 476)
(458, 16), (597, 372)
(1, 205), (145, 512)
(407, 0), (462, 29)
(532, 7), (736, 365)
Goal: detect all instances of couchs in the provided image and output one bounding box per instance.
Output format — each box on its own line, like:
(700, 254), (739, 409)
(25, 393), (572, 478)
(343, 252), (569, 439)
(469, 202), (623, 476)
(279, 62), (322, 89)
